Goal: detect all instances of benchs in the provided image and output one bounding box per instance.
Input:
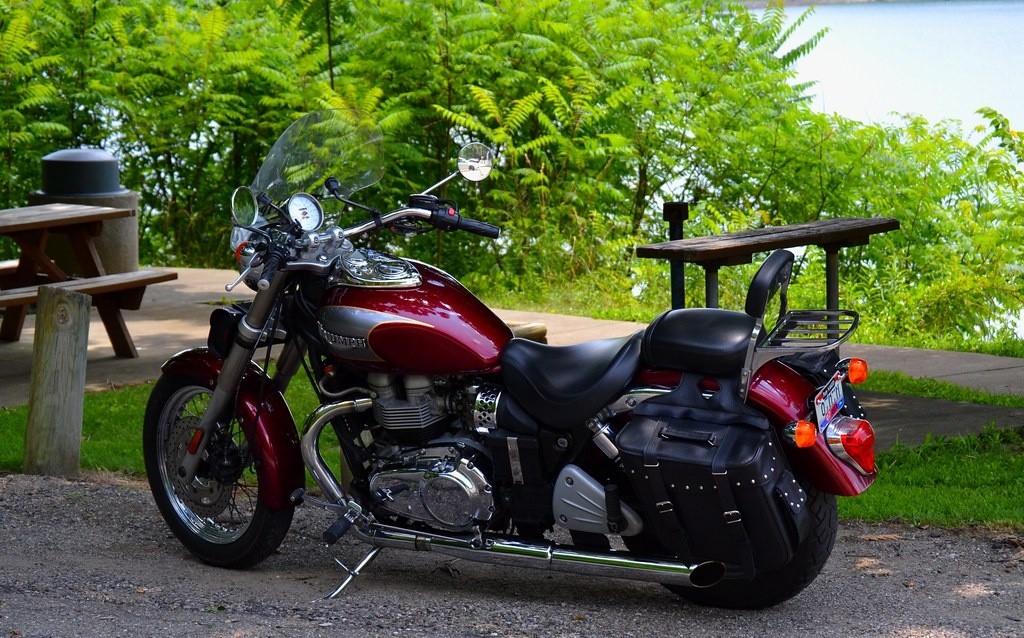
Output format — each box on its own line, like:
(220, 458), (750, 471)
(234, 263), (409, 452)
(0, 257), (179, 312)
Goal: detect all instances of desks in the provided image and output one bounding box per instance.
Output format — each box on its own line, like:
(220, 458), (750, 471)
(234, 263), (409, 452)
(0, 203), (140, 357)
(635, 216), (904, 377)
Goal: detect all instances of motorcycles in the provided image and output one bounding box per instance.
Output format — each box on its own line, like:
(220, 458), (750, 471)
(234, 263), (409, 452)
(142, 107), (877, 612)
